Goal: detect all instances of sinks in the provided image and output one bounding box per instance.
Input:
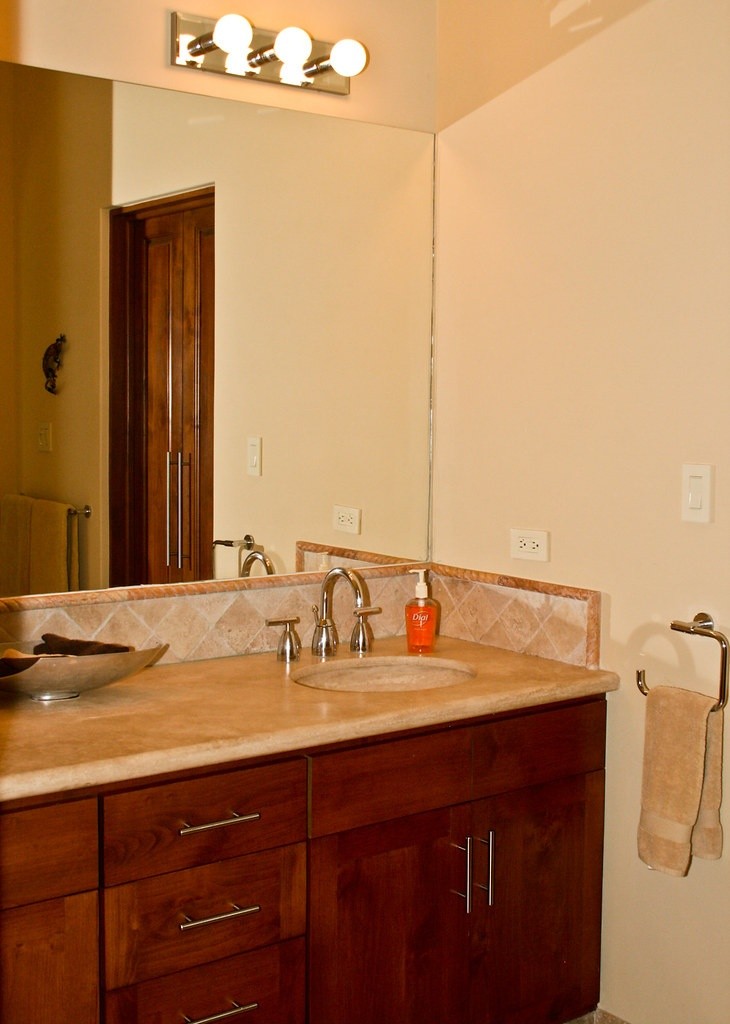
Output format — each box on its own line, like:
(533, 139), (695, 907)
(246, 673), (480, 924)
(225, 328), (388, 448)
(285, 649), (480, 696)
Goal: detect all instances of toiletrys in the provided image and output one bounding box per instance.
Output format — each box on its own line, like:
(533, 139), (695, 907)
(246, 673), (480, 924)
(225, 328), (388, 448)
(404, 564), (438, 655)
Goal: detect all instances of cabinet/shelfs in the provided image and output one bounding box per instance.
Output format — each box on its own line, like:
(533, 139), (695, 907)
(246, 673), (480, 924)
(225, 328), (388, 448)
(0, 697), (619, 1024)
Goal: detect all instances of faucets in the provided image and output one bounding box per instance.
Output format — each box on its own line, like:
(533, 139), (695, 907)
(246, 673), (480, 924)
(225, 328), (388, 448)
(311, 567), (374, 657)
(241, 550), (276, 580)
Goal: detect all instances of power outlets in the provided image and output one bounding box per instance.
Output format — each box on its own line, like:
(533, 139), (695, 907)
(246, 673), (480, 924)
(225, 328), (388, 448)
(332, 504), (361, 535)
(511, 530), (549, 563)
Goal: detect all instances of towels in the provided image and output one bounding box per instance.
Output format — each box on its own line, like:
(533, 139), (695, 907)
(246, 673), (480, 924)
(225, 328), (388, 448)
(0, 493), (31, 597)
(638, 683), (725, 879)
(30, 498), (82, 595)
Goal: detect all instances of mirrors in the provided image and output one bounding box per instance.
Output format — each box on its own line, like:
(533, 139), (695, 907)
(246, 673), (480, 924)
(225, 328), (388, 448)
(0, 58), (436, 601)
